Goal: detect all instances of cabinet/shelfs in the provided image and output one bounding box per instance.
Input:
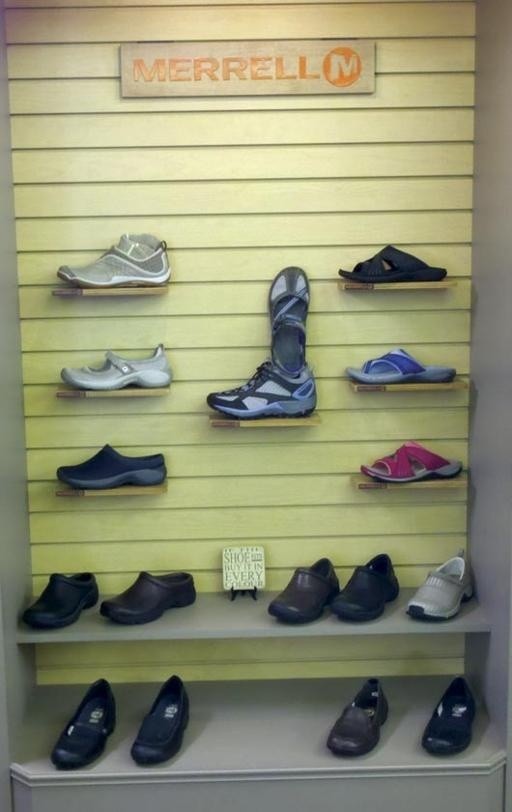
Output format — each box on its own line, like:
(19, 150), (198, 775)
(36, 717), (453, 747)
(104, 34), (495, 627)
(338, 281), (468, 492)
(49, 285), (170, 497)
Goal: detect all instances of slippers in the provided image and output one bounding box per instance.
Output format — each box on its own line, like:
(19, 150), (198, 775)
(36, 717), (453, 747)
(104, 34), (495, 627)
(337, 243), (448, 285)
(359, 440), (463, 484)
(345, 346), (457, 385)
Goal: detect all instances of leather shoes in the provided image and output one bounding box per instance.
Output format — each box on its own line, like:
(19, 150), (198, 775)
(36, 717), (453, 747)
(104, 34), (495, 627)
(47, 673), (118, 771)
(131, 670), (189, 769)
(327, 672), (389, 757)
(421, 673), (478, 756)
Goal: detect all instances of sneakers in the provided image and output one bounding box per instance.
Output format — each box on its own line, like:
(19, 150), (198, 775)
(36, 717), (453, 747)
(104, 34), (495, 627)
(205, 263), (317, 422)
(55, 232), (169, 291)
(21, 567), (197, 631)
(54, 442), (168, 491)
(267, 550), (401, 624)
(403, 550), (474, 623)
(59, 343), (174, 392)
